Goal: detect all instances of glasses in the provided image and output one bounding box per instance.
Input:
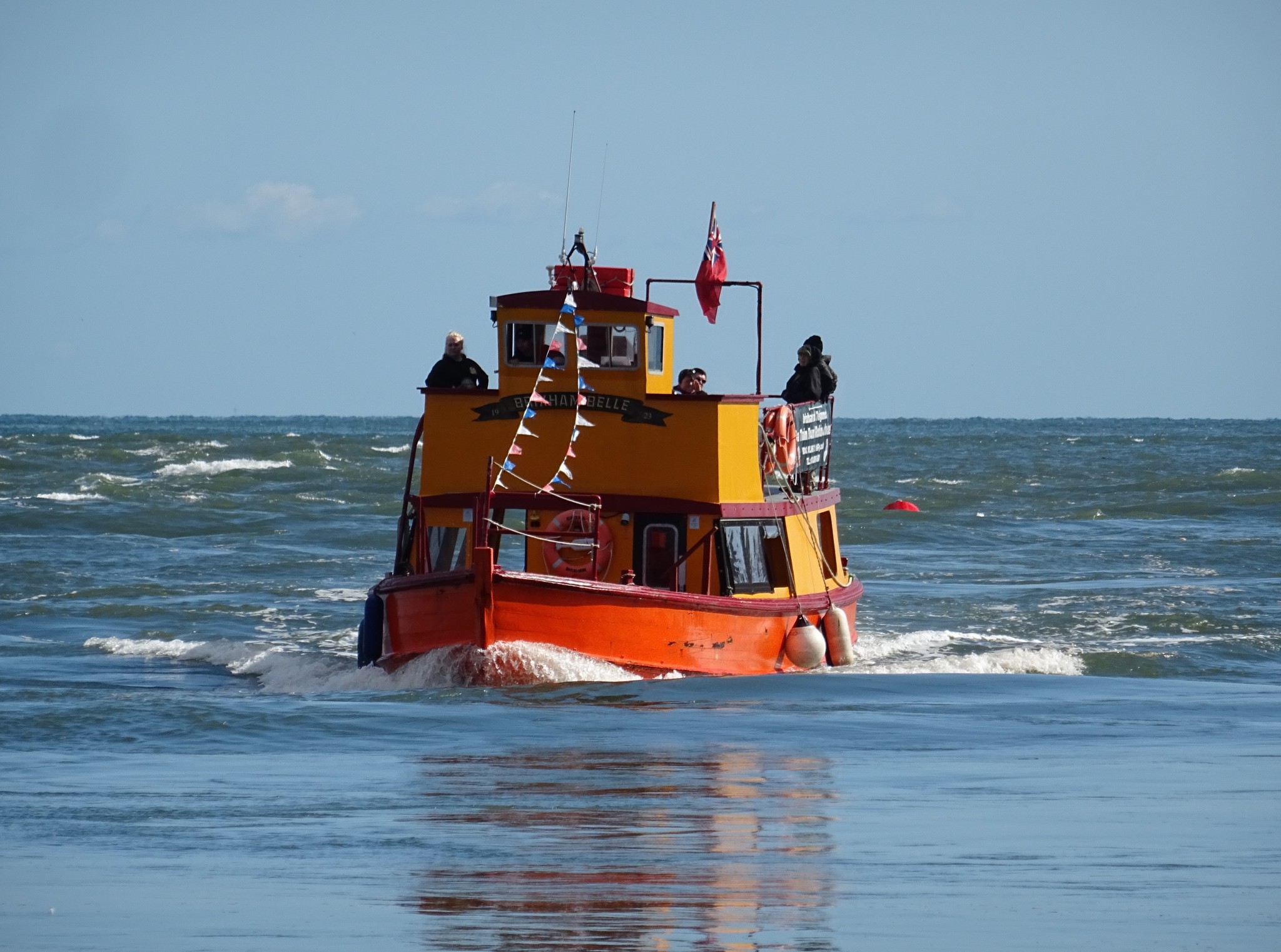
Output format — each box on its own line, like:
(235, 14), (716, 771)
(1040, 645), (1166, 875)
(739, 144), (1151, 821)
(696, 378), (706, 383)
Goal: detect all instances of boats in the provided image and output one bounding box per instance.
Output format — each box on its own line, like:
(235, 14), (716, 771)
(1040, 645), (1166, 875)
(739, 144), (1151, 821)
(358, 0), (866, 693)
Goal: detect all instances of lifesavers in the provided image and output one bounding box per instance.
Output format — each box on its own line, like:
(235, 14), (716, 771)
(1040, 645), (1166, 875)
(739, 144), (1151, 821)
(542, 508), (612, 580)
(762, 405), (797, 475)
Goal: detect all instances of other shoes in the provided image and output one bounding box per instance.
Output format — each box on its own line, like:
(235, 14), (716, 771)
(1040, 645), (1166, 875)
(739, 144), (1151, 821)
(779, 485), (800, 492)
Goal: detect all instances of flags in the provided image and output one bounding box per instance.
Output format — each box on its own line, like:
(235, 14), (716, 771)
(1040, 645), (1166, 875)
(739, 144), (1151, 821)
(695, 215), (728, 324)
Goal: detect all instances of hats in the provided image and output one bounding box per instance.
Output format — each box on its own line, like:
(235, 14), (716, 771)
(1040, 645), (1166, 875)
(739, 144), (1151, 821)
(798, 345), (812, 358)
(803, 335), (823, 353)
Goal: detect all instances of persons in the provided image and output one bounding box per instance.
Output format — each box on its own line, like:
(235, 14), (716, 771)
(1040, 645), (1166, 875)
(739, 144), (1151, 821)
(671, 367), (709, 395)
(425, 331), (488, 389)
(509, 328), (540, 364)
(781, 335), (837, 491)
